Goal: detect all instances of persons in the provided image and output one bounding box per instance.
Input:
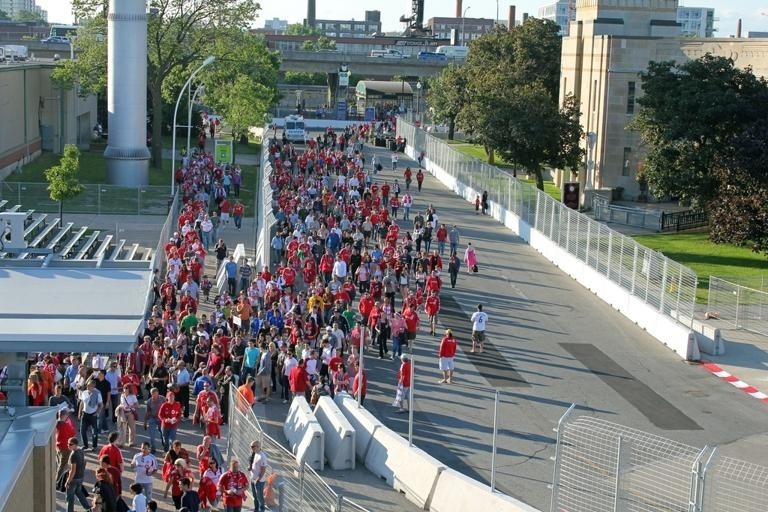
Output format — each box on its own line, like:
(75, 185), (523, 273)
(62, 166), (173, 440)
(140, 100), (490, 415)
(94, 122), (102, 142)
(26, 349), (270, 511)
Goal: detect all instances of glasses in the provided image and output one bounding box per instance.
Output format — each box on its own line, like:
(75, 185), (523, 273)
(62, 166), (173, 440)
(251, 446), (256, 448)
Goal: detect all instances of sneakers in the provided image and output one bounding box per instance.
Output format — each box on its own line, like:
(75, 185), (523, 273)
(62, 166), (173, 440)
(283, 400), (288, 403)
(438, 379), (453, 384)
(81, 446), (97, 452)
(181, 416), (189, 421)
(430, 331), (436, 336)
(258, 397), (270, 404)
(151, 447), (156, 454)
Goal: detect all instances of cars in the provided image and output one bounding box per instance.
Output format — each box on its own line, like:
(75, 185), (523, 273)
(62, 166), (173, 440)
(41, 36), (69, 45)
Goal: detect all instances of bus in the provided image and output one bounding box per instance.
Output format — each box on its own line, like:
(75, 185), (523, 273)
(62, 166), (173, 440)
(416, 51), (447, 61)
(49, 24), (103, 41)
(435, 46), (470, 58)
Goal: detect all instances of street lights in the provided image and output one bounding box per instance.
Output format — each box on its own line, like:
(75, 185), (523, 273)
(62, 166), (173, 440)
(461, 6), (470, 46)
(170, 54), (216, 197)
(186, 81), (204, 157)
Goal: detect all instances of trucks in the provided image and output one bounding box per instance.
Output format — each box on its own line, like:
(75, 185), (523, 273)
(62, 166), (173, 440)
(370, 48), (404, 58)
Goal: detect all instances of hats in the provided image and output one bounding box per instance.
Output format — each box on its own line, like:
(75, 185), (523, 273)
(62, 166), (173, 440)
(199, 362), (206, 368)
(333, 309), (340, 313)
(195, 251), (201, 255)
(170, 238), (176, 242)
(400, 355), (407, 359)
(167, 392), (174, 397)
(445, 329), (452, 334)
(326, 326), (332, 331)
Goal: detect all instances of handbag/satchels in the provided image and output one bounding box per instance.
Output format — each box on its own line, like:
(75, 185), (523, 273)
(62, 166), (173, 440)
(132, 411), (138, 421)
(398, 332), (408, 344)
(386, 326), (391, 339)
(310, 392), (317, 404)
(472, 265), (478, 272)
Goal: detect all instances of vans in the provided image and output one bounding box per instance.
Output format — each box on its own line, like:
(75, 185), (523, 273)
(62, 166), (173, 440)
(283, 114), (304, 141)
